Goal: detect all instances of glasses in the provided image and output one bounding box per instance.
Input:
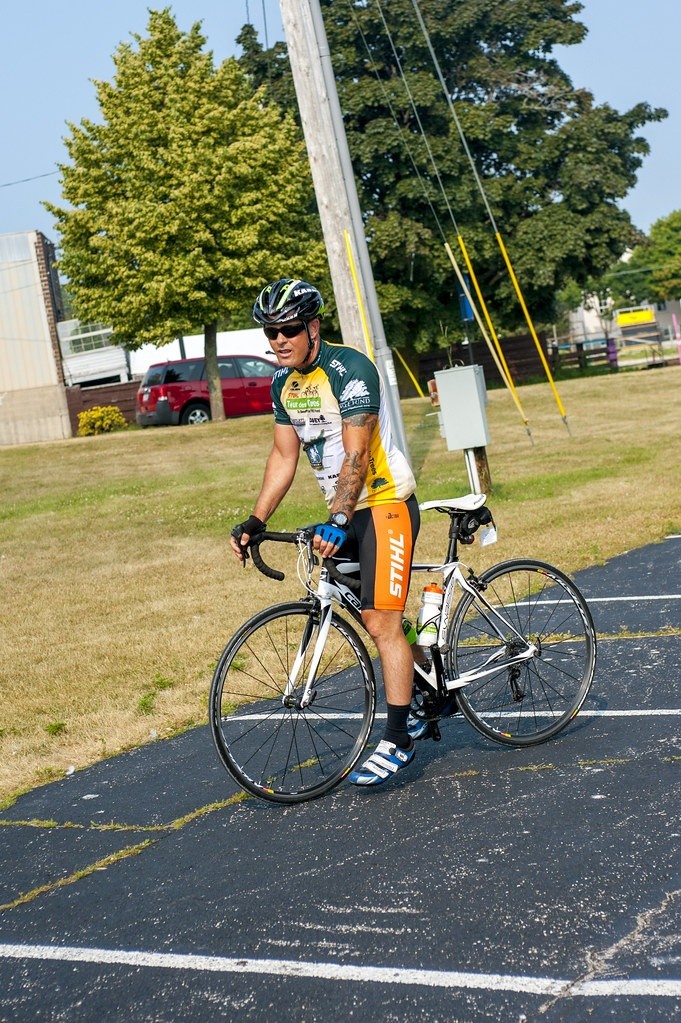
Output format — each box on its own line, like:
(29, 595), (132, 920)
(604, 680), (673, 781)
(263, 324), (309, 341)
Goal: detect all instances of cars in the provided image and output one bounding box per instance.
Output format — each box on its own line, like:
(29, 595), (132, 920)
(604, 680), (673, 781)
(137, 354), (280, 425)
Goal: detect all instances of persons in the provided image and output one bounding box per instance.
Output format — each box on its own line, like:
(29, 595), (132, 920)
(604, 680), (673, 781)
(230, 278), (432, 788)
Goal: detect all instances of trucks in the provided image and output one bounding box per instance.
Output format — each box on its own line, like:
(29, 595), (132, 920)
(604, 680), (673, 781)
(610, 305), (661, 346)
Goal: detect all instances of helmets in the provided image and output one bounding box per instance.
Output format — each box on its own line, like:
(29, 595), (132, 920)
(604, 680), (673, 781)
(252, 278), (324, 323)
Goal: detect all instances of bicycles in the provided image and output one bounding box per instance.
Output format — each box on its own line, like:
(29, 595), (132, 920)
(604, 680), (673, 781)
(208, 493), (598, 807)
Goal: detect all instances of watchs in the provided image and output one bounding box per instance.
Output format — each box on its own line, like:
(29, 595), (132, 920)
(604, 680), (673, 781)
(329, 511), (349, 527)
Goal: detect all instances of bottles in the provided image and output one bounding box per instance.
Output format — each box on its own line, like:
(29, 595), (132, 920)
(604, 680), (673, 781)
(416, 582), (445, 648)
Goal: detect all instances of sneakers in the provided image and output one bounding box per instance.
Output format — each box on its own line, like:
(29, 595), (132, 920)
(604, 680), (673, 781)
(347, 734), (415, 786)
(405, 700), (430, 742)
